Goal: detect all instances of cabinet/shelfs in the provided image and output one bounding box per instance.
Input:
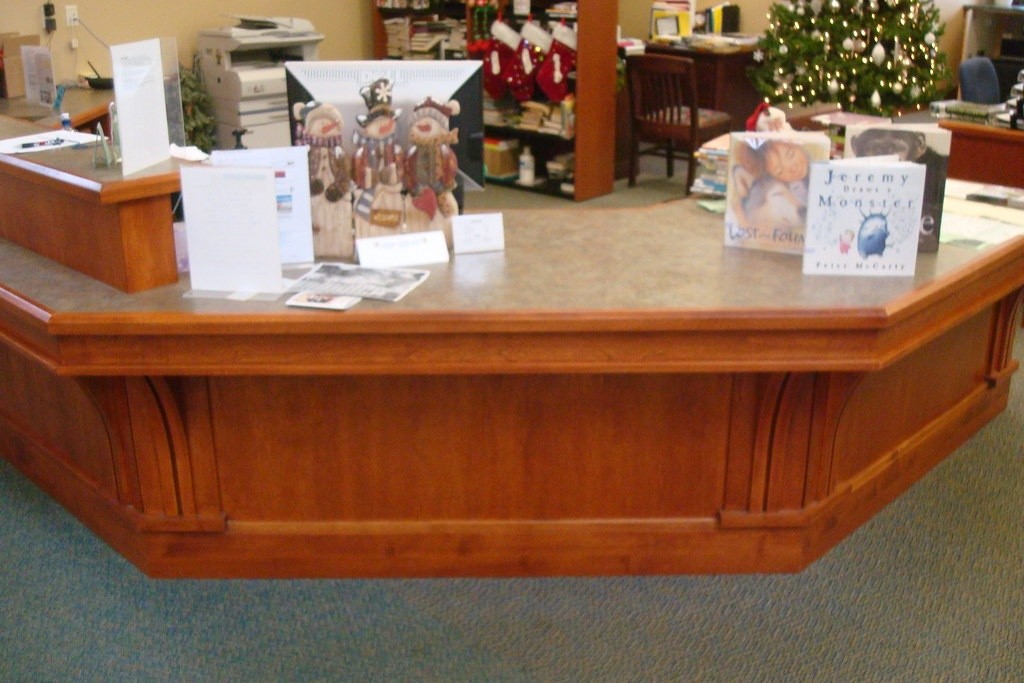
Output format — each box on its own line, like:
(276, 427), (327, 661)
(371, 0), (617, 204)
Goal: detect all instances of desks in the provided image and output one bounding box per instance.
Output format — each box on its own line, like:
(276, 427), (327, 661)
(640, 35), (765, 131)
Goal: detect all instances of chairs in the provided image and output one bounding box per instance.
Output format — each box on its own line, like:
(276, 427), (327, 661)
(959, 56), (1000, 106)
(625, 53), (732, 197)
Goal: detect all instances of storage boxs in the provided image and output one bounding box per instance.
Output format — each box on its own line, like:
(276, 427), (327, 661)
(810, 113), (892, 159)
